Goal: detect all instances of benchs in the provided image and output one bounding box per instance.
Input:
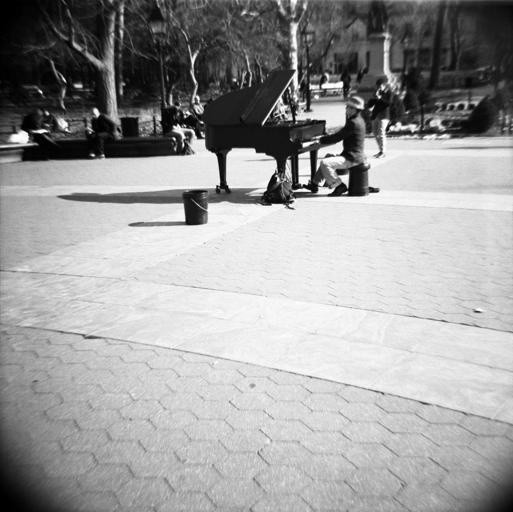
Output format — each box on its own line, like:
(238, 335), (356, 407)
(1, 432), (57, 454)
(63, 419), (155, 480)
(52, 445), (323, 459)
(0, 135), (175, 160)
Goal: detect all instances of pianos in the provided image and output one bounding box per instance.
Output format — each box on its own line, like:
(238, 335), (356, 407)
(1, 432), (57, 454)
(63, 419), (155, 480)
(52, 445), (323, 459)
(202, 70), (326, 193)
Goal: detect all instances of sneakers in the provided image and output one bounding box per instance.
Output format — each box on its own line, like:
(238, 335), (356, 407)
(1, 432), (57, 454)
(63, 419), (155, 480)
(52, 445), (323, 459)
(196, 125), (205, 138)
(373, 152), (385, 158)
(328, 183), (347, 196)
(303, 181), (318, 193)
(89, 153), (105, 158)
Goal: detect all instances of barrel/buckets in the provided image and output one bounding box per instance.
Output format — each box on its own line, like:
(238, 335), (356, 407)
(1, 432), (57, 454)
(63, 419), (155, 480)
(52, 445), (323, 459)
(183, 188), (209, 223)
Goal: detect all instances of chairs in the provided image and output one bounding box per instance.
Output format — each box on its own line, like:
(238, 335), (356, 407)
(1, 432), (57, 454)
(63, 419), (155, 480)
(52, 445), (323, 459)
(189, 109), (205, 136)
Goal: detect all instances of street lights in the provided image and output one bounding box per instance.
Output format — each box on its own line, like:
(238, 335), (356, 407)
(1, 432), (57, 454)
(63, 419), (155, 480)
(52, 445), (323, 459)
(301, 23), (315, 113)
(146, 9), (170, 135)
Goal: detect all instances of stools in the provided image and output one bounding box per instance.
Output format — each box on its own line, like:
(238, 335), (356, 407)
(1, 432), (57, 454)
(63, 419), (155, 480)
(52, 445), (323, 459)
(347, 164), (370, 195)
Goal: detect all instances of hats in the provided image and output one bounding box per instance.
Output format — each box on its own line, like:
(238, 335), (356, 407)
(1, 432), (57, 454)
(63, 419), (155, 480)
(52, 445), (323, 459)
(346, 96), (365, 110)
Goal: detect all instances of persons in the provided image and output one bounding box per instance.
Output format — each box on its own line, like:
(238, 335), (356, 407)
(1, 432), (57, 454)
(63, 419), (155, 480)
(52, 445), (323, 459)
(160, 94), (214, 155)
(367, 79), (394, 158)
(405, 56), (419, 121)
(84, 107), (122, 158)
(19, 110), (73, 160)
(299, 63), (368, 98)
(302, 96), (366, 196)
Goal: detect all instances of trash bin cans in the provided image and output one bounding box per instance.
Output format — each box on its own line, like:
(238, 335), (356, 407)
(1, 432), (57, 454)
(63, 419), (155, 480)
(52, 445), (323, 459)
(120, 117), (138, 136)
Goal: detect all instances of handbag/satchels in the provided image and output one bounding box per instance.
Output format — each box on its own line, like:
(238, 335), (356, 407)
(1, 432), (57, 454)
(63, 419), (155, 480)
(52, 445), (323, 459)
(182, 138), (195, 154)
(264, 171), (295, 203)
